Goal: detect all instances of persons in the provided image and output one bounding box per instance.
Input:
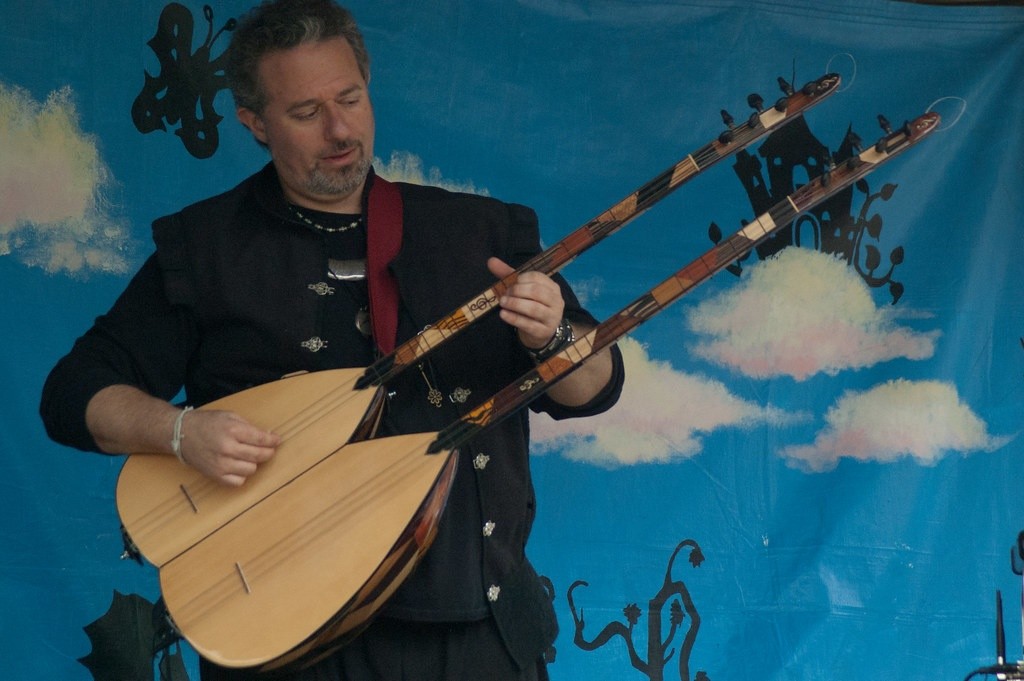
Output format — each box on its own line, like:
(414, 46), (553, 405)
(41, 0), (627, 681)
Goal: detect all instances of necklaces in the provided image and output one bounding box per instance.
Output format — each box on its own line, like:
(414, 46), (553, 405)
(281, 195), (363, 234)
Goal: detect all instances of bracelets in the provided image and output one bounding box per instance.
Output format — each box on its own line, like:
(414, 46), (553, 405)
(528, 317), (576, 363)
(172, 405), (192, 468)
